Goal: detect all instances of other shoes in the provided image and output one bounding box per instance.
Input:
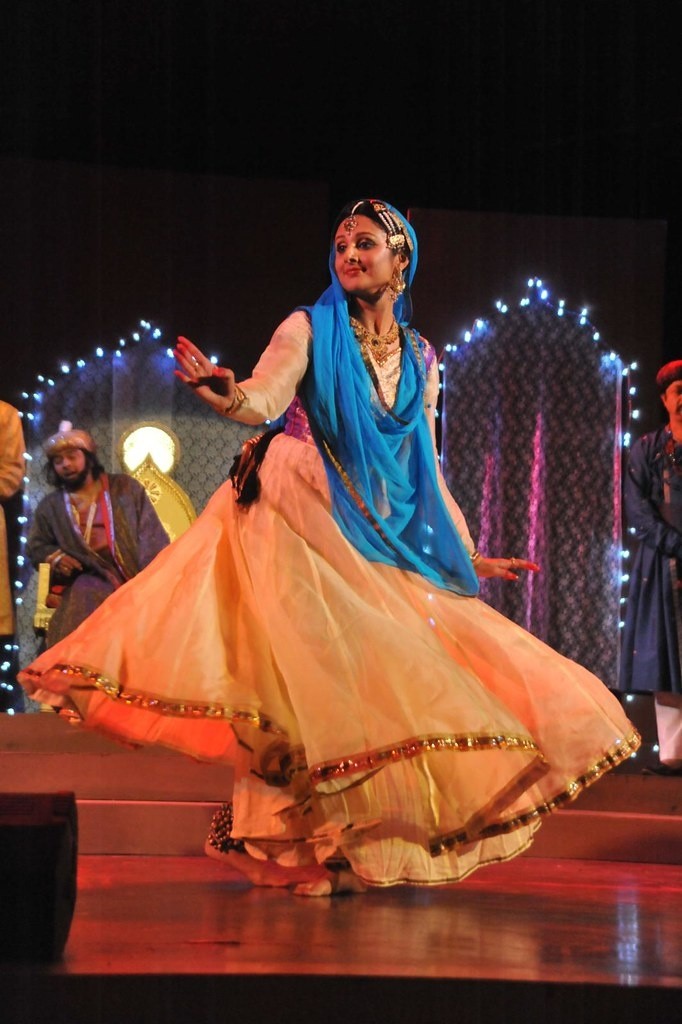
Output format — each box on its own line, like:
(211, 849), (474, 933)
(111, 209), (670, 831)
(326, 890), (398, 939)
(39, 703), (55, 713)
(641, 763), (682, 776)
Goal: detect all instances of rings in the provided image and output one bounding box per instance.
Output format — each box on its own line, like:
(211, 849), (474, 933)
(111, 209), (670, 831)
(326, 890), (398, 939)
(512, 557), (516, 567)
(64, 567), (68, 570)
(194, 362), (198, 368)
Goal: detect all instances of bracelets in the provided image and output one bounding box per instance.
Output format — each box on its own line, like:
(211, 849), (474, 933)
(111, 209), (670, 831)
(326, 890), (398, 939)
(471, 552), (482, 567)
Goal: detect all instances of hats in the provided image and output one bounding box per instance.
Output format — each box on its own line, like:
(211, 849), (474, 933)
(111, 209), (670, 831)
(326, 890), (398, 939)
(43, 429), (96, 459)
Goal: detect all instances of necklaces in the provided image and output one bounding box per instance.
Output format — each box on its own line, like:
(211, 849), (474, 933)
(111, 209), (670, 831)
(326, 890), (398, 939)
(70, 490), (98, 545)
(350, 315), (399, 356)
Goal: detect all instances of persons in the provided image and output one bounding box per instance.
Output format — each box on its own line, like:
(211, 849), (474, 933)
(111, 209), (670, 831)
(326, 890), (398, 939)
(0, 400), (26, 711)
(17, 198), (640, 898)
(24, 429), (172, 652)
(623, 360), (682, 775)
